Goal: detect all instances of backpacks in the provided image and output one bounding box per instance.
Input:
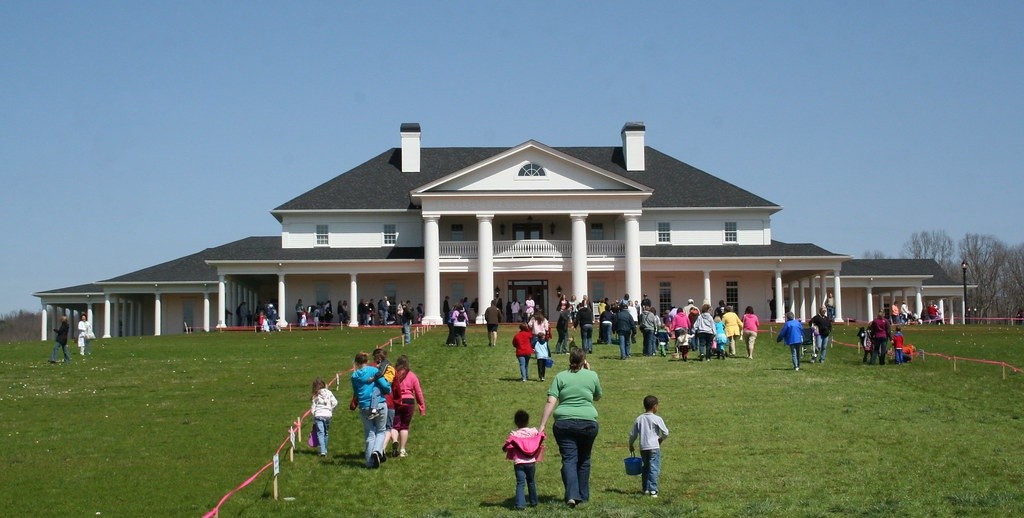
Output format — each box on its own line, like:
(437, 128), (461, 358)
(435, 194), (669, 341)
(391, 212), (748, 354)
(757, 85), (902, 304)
(456, 309), (464, 322)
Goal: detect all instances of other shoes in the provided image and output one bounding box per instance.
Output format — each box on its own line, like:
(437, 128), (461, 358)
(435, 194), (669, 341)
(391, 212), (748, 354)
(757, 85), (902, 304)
(392, 441), (399, 456)
(795, 368), (799, 372)
(463, 341), (466, 348)
(523, 380), (525, 382)
(700, 354), (705, 361)
(541, 377), (545, 381)
(567, 499), (574, 506)
(646, 490), (658, 498)
(322, 453), (326, 458)
(400, 450), (409, 458)
(368, 412), (379, 420)
(372, 451), (381, 469)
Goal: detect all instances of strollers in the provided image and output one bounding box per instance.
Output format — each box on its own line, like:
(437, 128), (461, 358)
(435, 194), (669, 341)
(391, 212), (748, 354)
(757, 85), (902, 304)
(709, 338), (728, 358)
(908, 311), (921, 325)
(800, 320), (819, 359)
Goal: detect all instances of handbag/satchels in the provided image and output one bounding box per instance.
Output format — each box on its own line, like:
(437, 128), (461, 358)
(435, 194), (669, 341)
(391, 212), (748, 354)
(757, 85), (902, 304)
(308, 423), (319, 447)
(712, 338), (717, 350)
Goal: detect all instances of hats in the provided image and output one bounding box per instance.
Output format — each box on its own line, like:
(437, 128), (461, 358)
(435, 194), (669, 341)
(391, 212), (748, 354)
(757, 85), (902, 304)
(688, 299), (694, 304)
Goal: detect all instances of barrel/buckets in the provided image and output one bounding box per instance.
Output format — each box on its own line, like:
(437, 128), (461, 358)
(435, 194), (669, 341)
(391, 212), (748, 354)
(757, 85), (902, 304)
(624, 449), (642, 475)
(545, 357), (554, 367)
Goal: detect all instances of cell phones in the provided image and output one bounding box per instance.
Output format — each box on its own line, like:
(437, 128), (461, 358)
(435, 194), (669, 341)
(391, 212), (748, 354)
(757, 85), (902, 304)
(584, 359), (587, 369)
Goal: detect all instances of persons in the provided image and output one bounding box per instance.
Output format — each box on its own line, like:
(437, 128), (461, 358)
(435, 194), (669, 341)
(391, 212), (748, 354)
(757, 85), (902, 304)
(47, 315), (70, 364)
(390, 354), (426, 458)
(629, 395), (668, 498)
(310, 379), (338, 457)
(295, 294), (944, 383)
(236, 300), (283, 336)
(502, 410), (546, 510)
(350, 353), (392, 469)
(367, 350), (396, 420)
(78, 314), (93, 356)
(538, 347), (603, 508)
(350, 349), (397, 463)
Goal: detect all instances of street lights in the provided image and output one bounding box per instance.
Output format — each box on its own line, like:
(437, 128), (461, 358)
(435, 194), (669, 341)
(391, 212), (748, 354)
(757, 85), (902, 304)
(962, 259), (968, 324)
(967, 308), (971, 324)
(974, 308), (977, 323)
